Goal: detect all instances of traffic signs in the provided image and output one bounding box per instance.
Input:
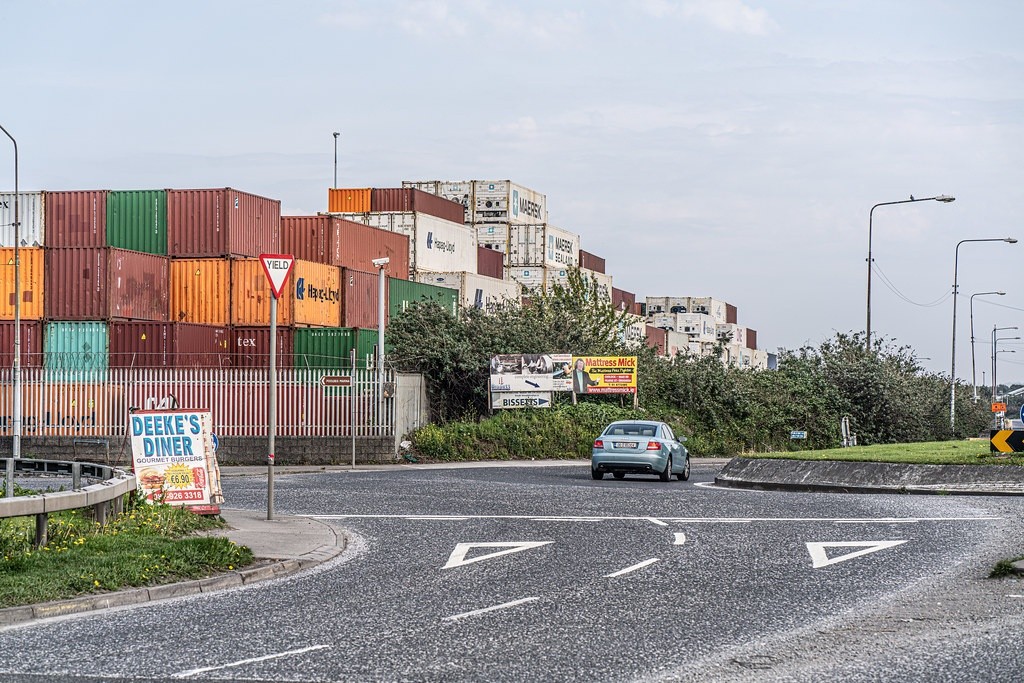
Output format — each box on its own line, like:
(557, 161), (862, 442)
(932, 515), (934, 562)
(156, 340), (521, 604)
(319, 375), (352, 387)
(989, 430), (1024, 454)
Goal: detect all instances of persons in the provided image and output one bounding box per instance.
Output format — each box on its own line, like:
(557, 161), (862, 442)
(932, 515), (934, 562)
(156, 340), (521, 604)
(573, 358), (601, 393)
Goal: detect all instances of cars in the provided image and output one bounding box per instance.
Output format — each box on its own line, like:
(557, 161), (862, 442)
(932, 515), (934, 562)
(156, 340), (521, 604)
(591, 420), (690, 481)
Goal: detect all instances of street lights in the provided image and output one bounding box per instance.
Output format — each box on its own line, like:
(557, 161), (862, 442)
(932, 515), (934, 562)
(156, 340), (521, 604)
(866, 194), (955, 354)
(995, 350), (1015, 398)
(970, 292), (1007, 405)
(948, 237), (1019, 433)
(994, 336), (1021, 402)
(990, 327), (1019, 405)
